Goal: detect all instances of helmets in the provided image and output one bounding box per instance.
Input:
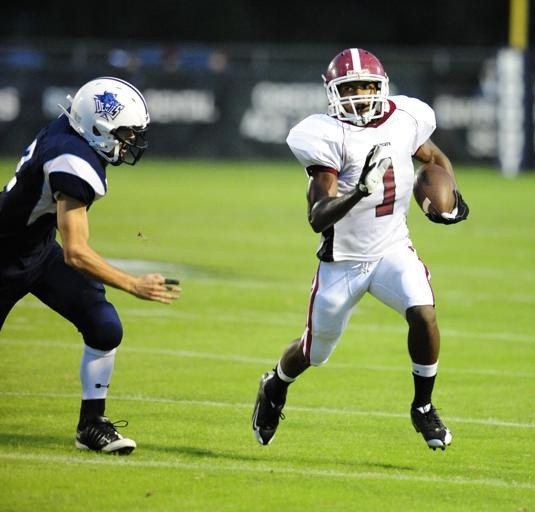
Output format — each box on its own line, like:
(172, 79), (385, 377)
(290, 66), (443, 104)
(322, 48), (389, 125)
(58, 76), (150, 166)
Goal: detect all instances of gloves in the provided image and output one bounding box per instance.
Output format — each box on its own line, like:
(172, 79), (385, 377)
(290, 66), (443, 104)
(359, 145), (392, 196)
(426, 192), (469, 224)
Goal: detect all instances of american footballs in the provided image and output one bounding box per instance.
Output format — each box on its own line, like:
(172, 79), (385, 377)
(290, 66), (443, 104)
(413, 163), (457, 216)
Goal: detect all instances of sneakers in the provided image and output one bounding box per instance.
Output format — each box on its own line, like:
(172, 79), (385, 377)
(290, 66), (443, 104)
(253, 371), (286, 445)
(75, 417), (136, 455)
(411, 403), (452, 450)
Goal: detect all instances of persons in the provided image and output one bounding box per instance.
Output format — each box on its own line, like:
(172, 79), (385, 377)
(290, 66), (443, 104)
(250, 46), (471, 454)
(0, 72), (183, 456)
